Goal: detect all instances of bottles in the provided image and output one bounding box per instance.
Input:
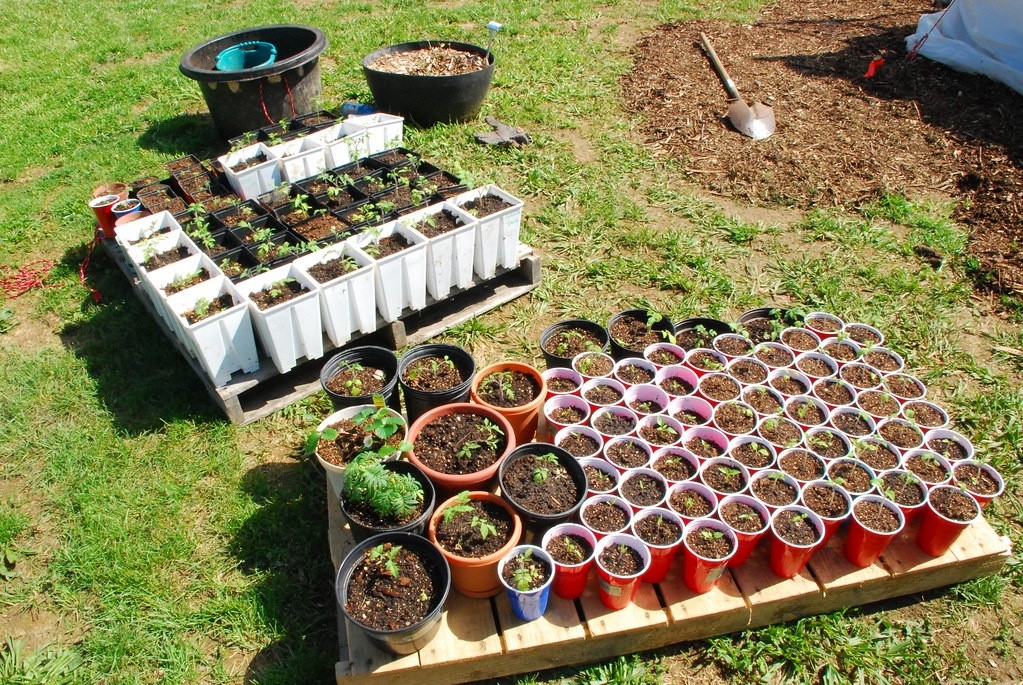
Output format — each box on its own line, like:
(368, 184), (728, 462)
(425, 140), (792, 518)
(341, 102), (375, 117)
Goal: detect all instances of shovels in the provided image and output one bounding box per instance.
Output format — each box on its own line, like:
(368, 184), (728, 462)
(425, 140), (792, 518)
(698, 31), (776, 140)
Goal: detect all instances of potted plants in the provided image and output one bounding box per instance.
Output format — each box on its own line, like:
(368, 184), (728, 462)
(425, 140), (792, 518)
(90, 39), (1007, 659)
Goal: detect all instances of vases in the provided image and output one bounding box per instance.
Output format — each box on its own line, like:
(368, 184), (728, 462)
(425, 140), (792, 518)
(215, 41), (278, 71)
(179, 23), (329, 138)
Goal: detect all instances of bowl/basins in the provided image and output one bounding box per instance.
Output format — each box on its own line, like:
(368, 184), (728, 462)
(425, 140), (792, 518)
(361, 40), (496, 129)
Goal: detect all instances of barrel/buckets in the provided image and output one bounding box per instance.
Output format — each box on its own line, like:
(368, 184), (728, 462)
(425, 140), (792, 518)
(216, 42), (277, 72)
(178, 24), (327, 146)
